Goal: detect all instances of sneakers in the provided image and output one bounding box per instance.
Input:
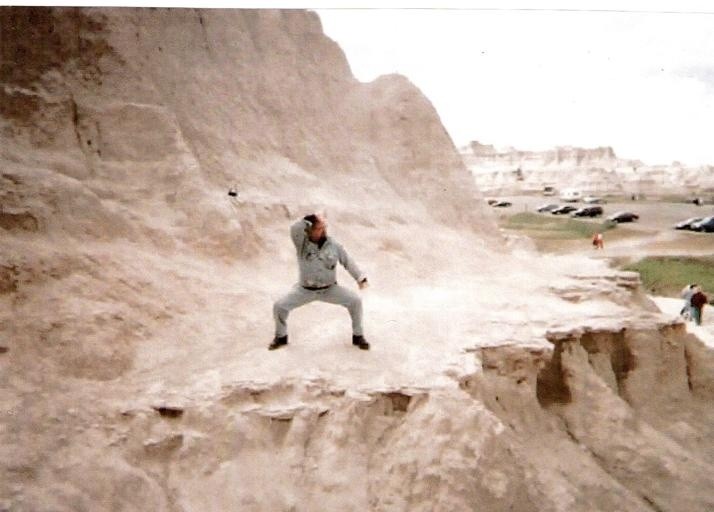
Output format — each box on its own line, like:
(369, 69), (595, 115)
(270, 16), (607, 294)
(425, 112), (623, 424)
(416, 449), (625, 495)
(266, 334), (290, 352)
(351, 334), (370, 350)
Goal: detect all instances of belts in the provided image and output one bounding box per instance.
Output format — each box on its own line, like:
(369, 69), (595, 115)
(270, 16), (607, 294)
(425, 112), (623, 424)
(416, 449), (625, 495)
(302, 285), (330, 291)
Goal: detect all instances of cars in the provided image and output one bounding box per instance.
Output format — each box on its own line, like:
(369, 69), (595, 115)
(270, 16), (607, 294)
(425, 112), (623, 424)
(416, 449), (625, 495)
(690, 215), (714, 232)
(494, 201), (512, 207)
(675, 217), (703, 230)
(606, 211), (639, 223)
(537, 185), (602, 217)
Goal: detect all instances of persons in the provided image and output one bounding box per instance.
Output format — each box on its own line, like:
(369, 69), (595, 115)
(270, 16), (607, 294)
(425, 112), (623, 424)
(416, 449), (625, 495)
(689, 285), (708, 324)
(266, 215), (370, 352)
(593, 233), (604, 250)
(680, 283), (699, 316)
